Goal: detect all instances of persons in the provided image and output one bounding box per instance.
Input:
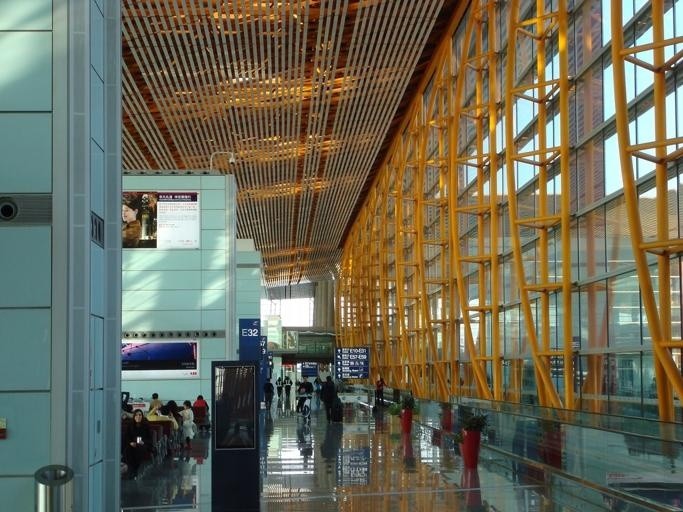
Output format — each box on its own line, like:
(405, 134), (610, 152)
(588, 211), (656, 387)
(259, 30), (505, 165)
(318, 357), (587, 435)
(121, 409), (155, 481)
(317, 376), (337, 423)
(296, 414), (307, 451)
(262, 409), (274, 442)
(120, 198), (142, 248)
(193, 396), (211, 431)
(296, 375), (314, 422)
(375, 377), (388, 406)
(274, 396), (292, 422)
(312, 375), (322, 394)
(263, 377), (274, 410)
(295, 387), (309, 414)
(158, 399), (184, 451)
(177, 400), (197, 450)
(146, 406), (183, 461)
(282, 376), (293, 397)
(275, 376), (283, 398)
(149, 393), (163, 413)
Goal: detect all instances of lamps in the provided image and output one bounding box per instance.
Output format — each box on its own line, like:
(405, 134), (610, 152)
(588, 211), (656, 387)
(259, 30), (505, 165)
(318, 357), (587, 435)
(210, 150), (235, 173)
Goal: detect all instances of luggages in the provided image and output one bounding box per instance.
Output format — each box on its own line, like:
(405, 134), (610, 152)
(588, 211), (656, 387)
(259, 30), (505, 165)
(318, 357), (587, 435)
(331, 398), (343, 422)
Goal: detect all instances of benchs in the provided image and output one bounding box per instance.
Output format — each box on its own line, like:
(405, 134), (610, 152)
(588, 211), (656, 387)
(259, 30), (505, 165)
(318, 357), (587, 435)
(115, 405), (209, 481)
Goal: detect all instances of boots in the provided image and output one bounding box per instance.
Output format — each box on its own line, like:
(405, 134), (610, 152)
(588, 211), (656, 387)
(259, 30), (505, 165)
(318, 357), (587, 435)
(185, 442), (192, 449)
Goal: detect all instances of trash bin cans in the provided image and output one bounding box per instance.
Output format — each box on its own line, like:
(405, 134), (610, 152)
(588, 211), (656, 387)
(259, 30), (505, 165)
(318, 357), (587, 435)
(34, 464), (74, 512)
